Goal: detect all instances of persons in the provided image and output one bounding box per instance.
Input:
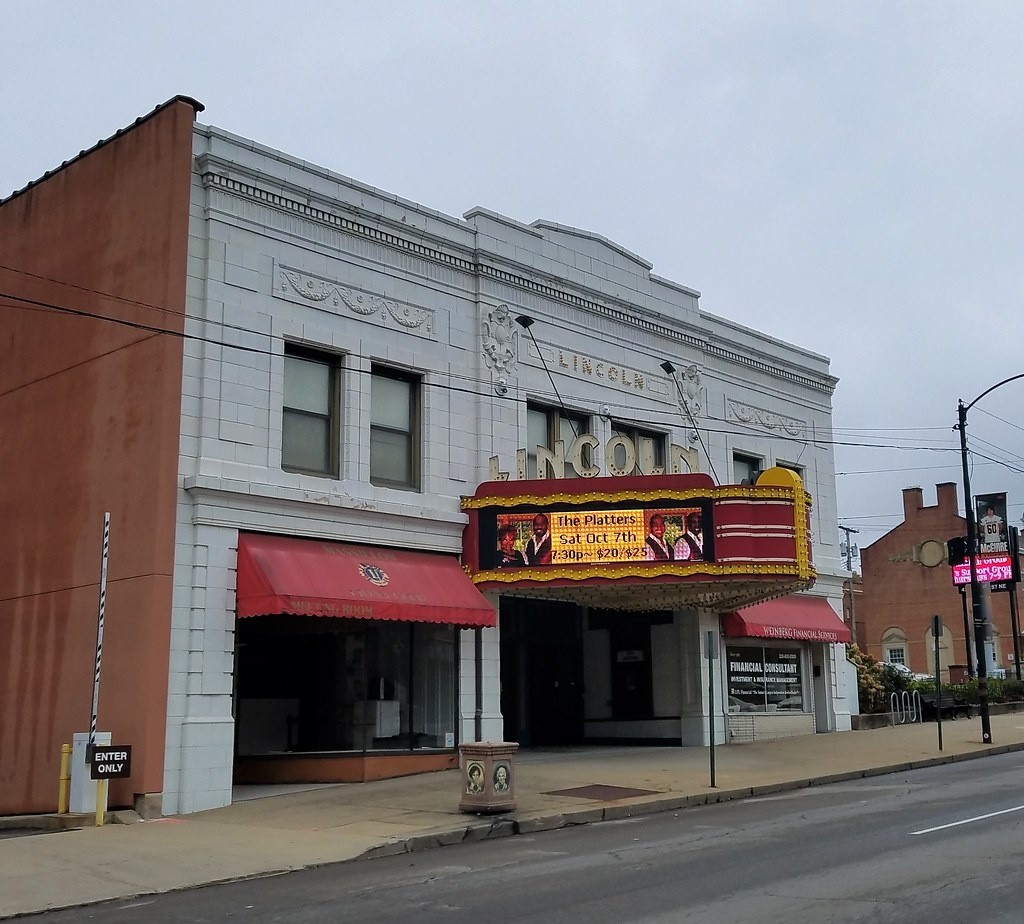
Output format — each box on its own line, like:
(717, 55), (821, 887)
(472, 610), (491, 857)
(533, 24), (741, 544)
(645, 512), (704, 560)
(979, 506), (1004, 545)
(466, 766), (485, 794)
(497, 514), (552, 567)
(495, 768), (507, 792)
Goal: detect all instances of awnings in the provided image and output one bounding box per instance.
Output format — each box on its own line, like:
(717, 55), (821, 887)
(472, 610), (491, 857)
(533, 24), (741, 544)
(237, 532), (497, 630)
(722, 595), (851, 642)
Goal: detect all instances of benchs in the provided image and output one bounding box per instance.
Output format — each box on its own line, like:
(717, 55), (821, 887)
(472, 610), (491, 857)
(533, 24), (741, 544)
(911, 692), (971, 721)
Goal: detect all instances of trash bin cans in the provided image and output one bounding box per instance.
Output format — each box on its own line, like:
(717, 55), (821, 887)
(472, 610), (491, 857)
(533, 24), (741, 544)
(458, 741), (520, 812)
(948, 664), (968, 685)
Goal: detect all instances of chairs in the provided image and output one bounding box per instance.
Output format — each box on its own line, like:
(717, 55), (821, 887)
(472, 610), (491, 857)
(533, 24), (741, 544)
(729, 696), (802, 712)
